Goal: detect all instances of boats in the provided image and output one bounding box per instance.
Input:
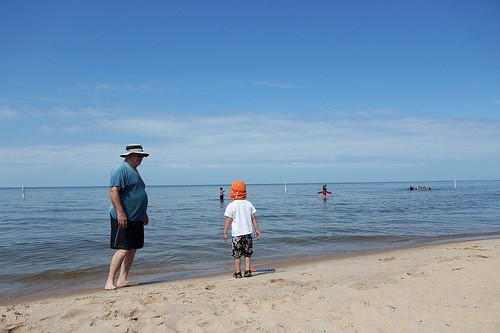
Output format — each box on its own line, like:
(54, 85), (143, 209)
(409, 185), (432, 191)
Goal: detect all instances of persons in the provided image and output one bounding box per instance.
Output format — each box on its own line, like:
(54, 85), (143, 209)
(409, 186), (427, 190)
(223, 180), (261, 277)
(104, 144), (150, 290)
(323, 184), (327, 197)
(220, 187), (226, 208)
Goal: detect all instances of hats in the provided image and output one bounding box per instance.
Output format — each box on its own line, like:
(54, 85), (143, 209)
(120, 144), (150, 158)
(229, 180), (246, 200)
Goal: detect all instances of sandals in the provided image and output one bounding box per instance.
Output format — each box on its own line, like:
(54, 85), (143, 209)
(233, 271), (242, 278)
(243, 269), (252, 277)
